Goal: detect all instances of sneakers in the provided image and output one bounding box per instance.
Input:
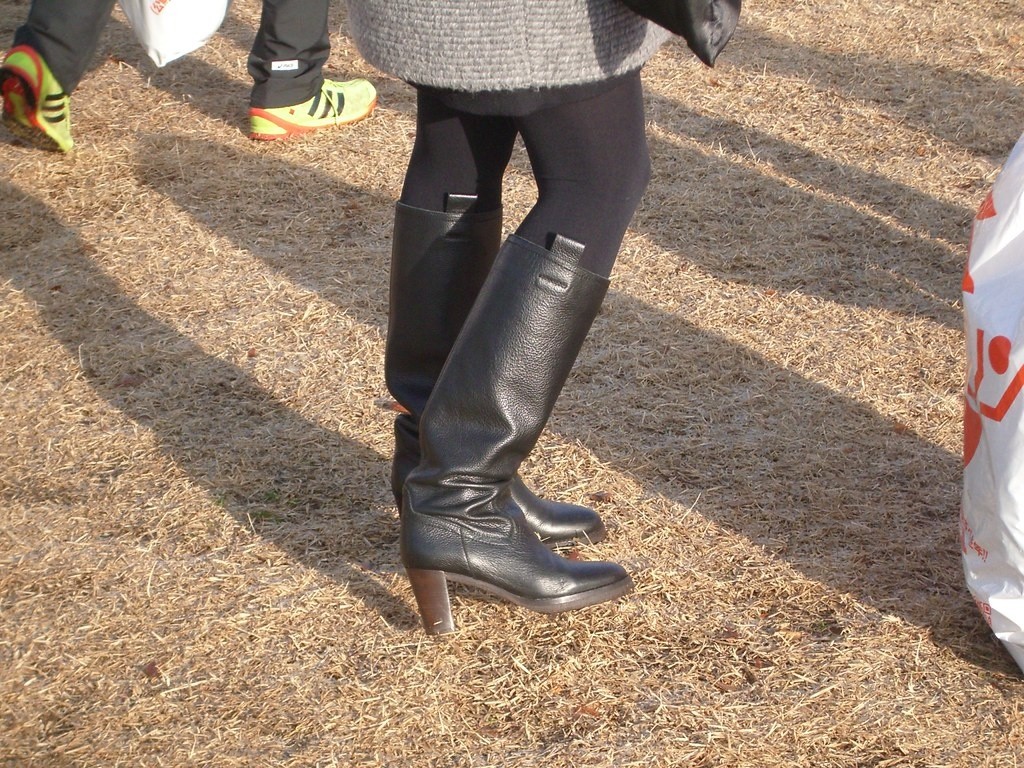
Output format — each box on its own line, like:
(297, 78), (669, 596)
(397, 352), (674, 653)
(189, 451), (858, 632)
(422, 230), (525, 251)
(248, 77), (377, 140)
(0, 45), (74, 153)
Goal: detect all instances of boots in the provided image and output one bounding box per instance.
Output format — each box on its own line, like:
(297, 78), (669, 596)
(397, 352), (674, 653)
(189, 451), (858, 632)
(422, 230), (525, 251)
(384, 197), (606, 549)
(402, 234), (632, 636)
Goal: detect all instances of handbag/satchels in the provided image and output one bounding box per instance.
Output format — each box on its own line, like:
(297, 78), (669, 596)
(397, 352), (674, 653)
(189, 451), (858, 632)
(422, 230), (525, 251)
(960, 133), (1023, 670)
(619, 0), (743, 65)
(119, 0), (231, 69)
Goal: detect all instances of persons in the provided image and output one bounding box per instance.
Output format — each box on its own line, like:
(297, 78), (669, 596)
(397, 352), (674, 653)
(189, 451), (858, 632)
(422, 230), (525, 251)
(0, 0), (377, 151)
(337, 0), (686, 637)
